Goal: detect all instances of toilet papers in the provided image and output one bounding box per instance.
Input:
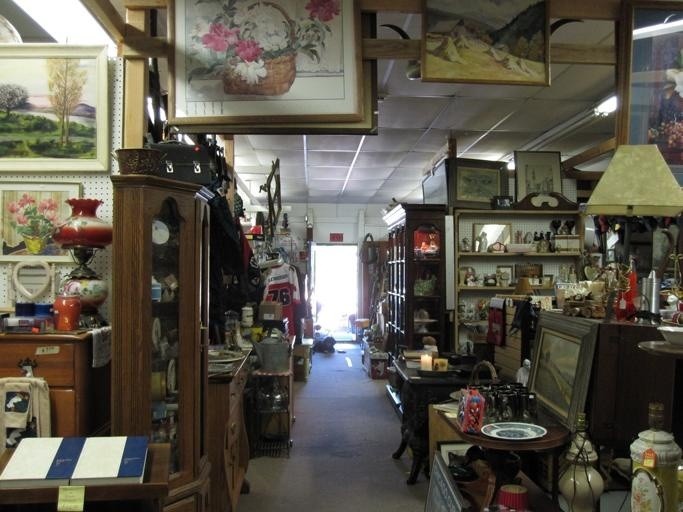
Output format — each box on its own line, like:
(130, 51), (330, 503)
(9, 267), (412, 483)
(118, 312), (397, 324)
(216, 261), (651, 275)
(241, 306), (254, 328)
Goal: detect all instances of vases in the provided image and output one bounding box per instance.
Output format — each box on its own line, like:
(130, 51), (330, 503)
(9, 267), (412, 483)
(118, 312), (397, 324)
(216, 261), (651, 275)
(53, 197), (112, 246)
(22, 233), (47, 254)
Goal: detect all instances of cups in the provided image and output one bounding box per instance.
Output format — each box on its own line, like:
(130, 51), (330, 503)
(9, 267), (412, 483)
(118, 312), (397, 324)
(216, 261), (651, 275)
(555, 235), (580, 253)
(15, 300), (52, 318)
(433, 358), (448, 372)
(469, 380), (539, 427)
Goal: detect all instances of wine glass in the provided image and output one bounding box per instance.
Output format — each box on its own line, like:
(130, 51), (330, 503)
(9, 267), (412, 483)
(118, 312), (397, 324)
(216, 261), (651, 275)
(552, 219), (576, 236)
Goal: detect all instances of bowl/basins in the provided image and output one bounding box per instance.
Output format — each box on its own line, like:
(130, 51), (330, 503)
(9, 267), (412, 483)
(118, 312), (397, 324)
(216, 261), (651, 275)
(656, 325), (683, 347)
(507, 243), (531, 253)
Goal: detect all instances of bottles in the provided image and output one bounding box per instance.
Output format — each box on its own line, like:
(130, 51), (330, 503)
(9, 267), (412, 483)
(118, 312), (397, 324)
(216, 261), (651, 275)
(242, 307), (254, 326)
(640, 271), (662, 319)
(534, 231), (552, 252)
(225, 308), (242, 349)
(478, 232), (488, 252)
(629, 400), (683, 512)
(54, 291), (82, 331)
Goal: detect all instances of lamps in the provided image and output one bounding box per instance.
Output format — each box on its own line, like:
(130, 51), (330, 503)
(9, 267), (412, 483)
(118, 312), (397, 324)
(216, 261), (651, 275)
(579, 144), (682, 320)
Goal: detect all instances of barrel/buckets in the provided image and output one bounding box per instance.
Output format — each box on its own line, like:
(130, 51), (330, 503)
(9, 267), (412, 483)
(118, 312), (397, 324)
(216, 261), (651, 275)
(369, 358), (390, 380)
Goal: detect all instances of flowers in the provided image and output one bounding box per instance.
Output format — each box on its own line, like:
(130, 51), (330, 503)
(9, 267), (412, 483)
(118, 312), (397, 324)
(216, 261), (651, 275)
(7, 191), (63, 239)
(186, 1), (343, 86)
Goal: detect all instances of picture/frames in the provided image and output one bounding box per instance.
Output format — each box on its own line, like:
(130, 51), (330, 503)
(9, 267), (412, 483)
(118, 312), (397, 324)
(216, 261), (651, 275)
(167, 10), (378, 135)
(613, 0), (682, 166)
(421, 1), (552, 87)
(528, 313), (598, 432)
(471, 222), (512, 253)
(0, 180), (84, 265)
(11, 258), (52, 300)
(448, 150), (563, 210)
(0, 41), (110, 172)
(166, 0), (366, 125)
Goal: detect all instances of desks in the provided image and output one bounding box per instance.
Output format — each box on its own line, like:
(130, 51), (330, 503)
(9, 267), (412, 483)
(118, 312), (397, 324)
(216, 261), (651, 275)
(389, 355), (492, 485)
(1, 443), (171, 511)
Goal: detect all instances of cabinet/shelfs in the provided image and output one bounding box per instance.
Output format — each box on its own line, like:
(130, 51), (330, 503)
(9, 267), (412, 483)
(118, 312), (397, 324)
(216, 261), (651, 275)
(110, 173), (212, 512)
(382, 202), (447, 369)
(0, 326), (112, 437)
(428, 398), (570, 512)
(539, 308), (683, 469)
(209, 330), (313, 511)
(493, 293), (556, 385)
(453, 208), (593, 360)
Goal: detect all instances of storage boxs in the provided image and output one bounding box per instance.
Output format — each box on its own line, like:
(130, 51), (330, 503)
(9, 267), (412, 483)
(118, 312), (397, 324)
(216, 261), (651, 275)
(363, 347), (389, 379)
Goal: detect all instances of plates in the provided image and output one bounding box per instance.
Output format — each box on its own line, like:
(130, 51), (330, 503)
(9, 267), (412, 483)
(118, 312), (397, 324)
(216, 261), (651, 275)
(209, 350), (247, 362)
(480, 420), (550, 443)
(153, 221), (169, 245)
(416, 370), (461, 378)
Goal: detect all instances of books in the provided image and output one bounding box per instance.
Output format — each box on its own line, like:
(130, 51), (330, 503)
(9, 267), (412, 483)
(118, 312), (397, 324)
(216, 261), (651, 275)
(0, 436), (150, 490)
